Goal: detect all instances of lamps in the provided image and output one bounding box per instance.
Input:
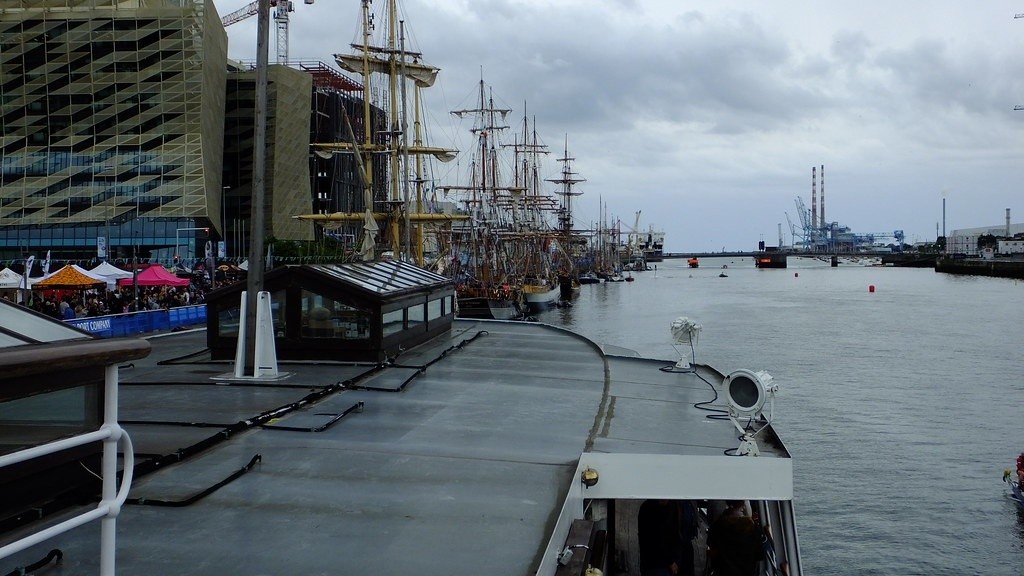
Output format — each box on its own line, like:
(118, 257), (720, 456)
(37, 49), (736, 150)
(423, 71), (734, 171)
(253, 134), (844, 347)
(723, 368), (779, 457)
(668, 314), (704, 368)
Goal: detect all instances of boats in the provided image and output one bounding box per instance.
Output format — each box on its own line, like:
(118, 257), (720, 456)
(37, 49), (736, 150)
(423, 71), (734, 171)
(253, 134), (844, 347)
(687, 255), (700, 269)
(753, 250), (789, 269)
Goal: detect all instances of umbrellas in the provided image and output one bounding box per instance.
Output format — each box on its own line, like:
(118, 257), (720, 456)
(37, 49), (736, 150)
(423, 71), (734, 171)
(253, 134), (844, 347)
(165, 260), (249, 277)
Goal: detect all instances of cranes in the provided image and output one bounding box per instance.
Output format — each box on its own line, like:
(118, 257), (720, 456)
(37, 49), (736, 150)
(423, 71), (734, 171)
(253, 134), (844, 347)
(784, 195), (858, 248)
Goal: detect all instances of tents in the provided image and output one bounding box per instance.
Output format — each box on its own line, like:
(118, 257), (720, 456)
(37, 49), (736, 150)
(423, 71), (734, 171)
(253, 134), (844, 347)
(0, 262), (193, 315)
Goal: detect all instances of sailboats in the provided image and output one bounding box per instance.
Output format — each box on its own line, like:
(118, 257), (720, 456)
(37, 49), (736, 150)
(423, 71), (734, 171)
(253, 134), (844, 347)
(293, 0), (665, 320)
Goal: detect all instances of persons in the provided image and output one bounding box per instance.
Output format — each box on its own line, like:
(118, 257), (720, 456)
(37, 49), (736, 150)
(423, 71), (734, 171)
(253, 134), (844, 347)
(638, 499), (698, 576)
(456, 281), (511, 298)
(1016, 453), (1024, 485)
(706, 500), (766, 576)
(2, 277), (245, 320)
